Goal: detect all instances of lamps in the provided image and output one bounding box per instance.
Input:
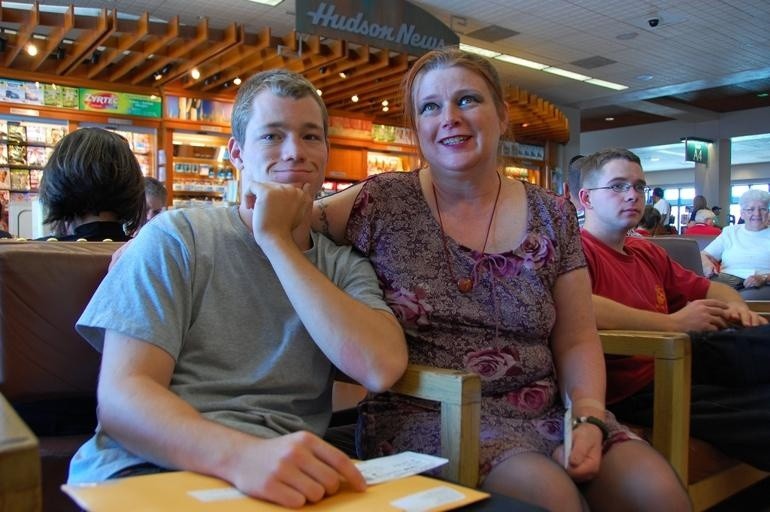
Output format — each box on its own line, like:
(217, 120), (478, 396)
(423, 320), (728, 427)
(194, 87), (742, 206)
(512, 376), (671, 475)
(316, 89), (321, 96)
(27, 46), (37, 58)
(191, 70), (200, 79)
(521, 122), (530, 129)
(351, 95), (358, 102)
(153, 72), (163, 81)
(233, 78), (241, 86)
(340, 72), (346, 79)
(381, 100), (390, 112)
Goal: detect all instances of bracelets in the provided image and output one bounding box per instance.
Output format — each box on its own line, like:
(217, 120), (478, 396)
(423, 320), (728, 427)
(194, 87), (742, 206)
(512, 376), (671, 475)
(572, 416), (610, 441)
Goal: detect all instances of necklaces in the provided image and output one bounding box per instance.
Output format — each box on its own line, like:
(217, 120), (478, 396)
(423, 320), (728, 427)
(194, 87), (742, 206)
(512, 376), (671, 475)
(432, 168), (502, 294)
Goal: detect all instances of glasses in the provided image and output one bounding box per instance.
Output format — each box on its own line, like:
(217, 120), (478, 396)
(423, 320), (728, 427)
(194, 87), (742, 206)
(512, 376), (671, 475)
(587, 183), (652, 193)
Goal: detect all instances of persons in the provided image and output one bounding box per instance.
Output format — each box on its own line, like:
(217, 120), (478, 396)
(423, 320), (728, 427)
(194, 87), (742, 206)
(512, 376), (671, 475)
(712, 206), (722, 226)
(67, 70), (408, 508)
(686, 209), (722, 236)
(140, 177), (168, 222)
(563, 157), (588, 227)
(652, 187), (670, 228)
(31, 128), (142, 242)
(578, 149), (770, 472)
(635, 207), (661, 238)
(0, 202), (12, 238)
(688, 195), (708, 223)
(313, 48), (694, 512)
(700, 190), (769, 301)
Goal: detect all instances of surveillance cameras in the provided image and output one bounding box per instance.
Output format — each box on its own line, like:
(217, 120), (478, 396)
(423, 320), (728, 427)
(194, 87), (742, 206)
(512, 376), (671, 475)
(645, 17), (662, 28)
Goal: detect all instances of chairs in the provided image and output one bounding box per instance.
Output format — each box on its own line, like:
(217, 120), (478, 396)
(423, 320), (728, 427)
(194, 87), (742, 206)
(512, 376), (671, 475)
(586, 235), (770, 511)
(333, 323), (692, 512)
(0, 241), (479, 512)
